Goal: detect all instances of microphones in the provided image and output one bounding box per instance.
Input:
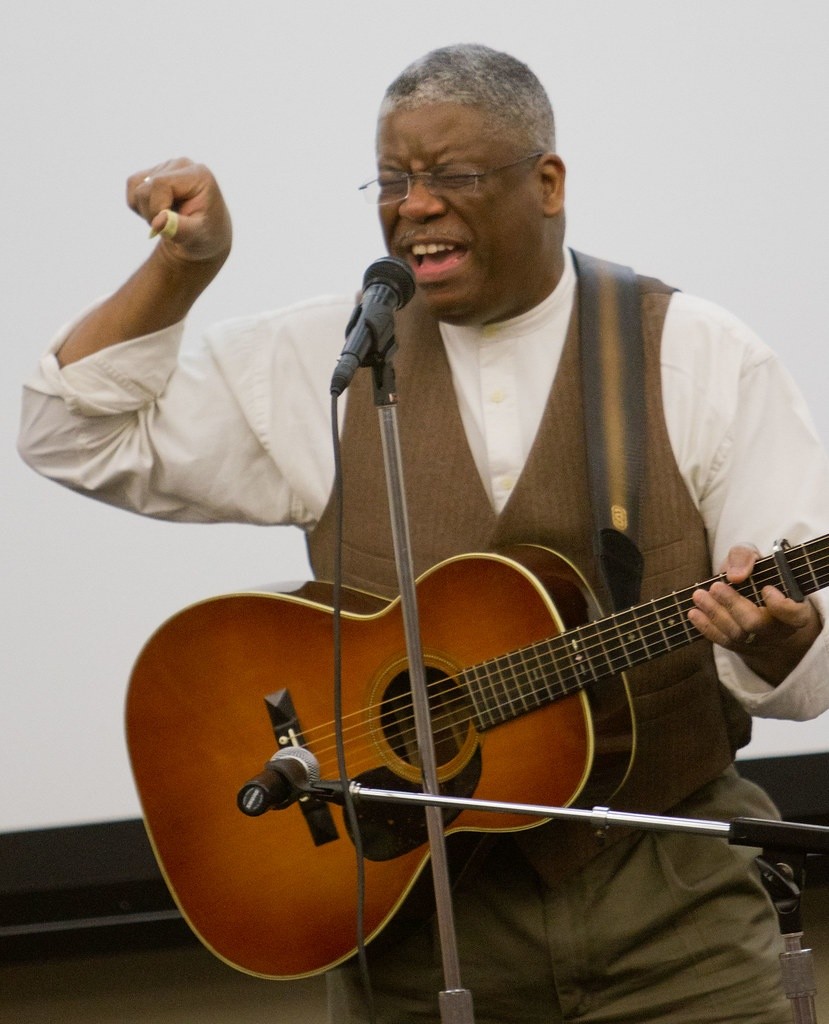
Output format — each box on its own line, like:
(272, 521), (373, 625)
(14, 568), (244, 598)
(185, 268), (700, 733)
(236, 747), (319, 816)
(330, 257), (416, 397)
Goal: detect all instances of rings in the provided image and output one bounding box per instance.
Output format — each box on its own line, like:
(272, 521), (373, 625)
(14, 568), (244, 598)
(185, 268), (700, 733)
(143, 177), (153, 186)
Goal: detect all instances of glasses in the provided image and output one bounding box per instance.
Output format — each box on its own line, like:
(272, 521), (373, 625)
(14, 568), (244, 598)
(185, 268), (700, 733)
(356, 148), (549, 207)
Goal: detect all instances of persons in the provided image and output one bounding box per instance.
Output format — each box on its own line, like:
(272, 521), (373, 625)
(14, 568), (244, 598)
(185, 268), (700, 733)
(18, 43), (829, 1024)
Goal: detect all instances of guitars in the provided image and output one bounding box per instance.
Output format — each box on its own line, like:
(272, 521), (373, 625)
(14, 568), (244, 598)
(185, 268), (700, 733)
(124, 534), (829, 983)
